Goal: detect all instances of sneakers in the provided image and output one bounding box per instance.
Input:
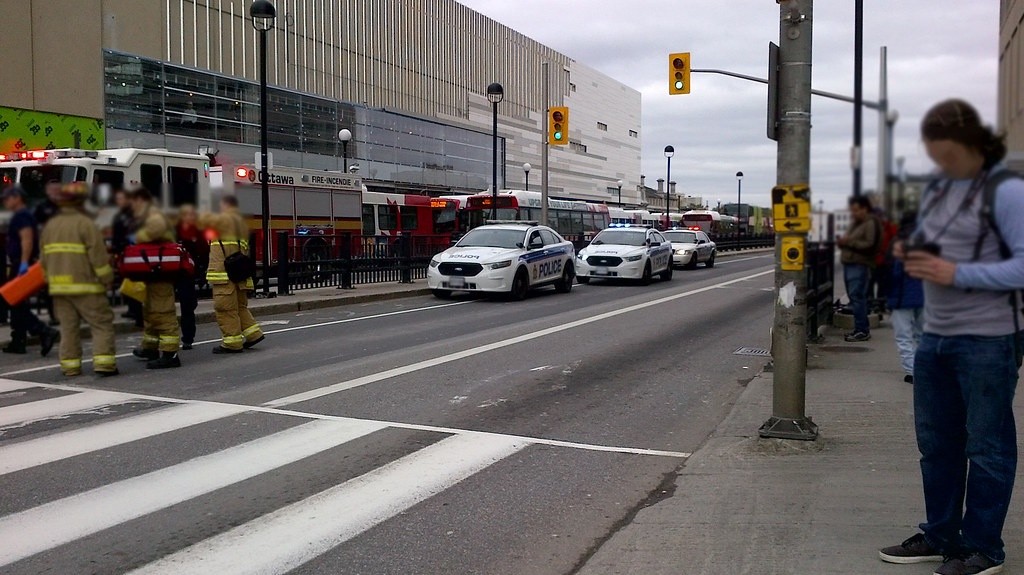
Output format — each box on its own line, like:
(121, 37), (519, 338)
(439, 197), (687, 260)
(878, 532), (949, 563)
(934, 545), (1003, 575)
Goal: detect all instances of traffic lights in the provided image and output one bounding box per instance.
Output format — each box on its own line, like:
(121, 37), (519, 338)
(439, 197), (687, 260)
(549, 107), (569, 145)
(669, 52), (690, 95)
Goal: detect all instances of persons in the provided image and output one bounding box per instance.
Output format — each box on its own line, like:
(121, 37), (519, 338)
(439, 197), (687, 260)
(0, 176), (210, 378)
(837, 193), (927, 383)
(878, 98), (1024, 575)
(195, 193), (266, 354)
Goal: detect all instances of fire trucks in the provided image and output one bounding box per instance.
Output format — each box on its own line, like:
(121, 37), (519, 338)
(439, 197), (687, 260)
(0, 144), (364, 287)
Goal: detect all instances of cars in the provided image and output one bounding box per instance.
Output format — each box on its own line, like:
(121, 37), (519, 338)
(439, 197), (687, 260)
(427, 220), (575, 302)
(573, 223), (675, 287)
(660, 227), (717, 270)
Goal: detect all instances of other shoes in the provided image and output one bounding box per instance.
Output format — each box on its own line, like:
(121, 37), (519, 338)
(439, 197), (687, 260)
(2, 345), (27, 354)
(40, 328), (58, 356)
(133, 347), (160, 360)
(121, 312), (135, 317)
(844, 328), (871, 342)
(97, 369), (119, 376)
(242, 334), (266, 349)
(146, 350), (181, 368)
(183, 342), (192, 350)
(213, 345), (242, 354)
(904, 374), (913, 384)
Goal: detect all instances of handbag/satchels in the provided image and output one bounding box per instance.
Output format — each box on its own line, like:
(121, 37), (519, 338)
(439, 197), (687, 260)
(224, 251), (256, 284)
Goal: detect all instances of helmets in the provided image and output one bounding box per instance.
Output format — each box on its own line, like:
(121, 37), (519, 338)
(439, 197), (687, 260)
(56, 183), (87, 207)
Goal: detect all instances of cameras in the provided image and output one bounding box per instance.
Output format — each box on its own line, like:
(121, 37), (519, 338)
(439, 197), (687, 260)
(902, 242), (941, 258)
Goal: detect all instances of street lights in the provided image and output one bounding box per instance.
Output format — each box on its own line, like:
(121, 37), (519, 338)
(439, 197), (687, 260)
(663, 145), (674, 230)
(339, 128), (352, 173)
(717, 199), (721, 212)
(487, 84), (504, 220)
(523, 163), (532, 191)
(887, 110), (905, 219)
(736, 171), (744, 250)
(249, 1), (277, 297)
(617, 180), (623, 208)
(818, 199), (824, 244)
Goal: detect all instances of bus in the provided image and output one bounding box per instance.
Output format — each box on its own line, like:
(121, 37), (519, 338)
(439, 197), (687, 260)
(469, 188), (611, 250)
(363, 182), (432, 260)
(432, 195), (476, 255)
(608, 206), (739, 242)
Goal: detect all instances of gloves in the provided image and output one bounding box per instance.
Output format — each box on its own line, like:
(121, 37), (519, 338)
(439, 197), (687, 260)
(18, 261), (28, 276)
